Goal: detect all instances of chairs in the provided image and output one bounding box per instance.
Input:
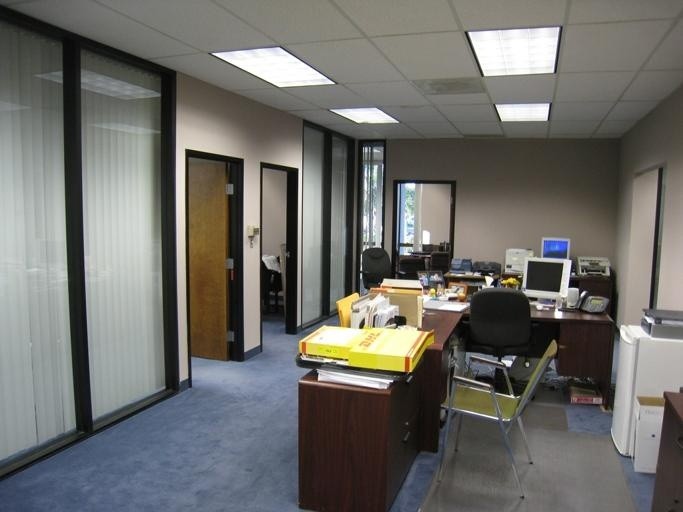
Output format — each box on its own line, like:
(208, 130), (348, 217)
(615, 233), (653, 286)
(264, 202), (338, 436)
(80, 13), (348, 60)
(441, 339), (565, 500)
(361, 248), (411, 296)
(398, 257), (427, 282)
(262, 251), (281, 318)
(428, 251), (448, 271)
(475, 289), (545, 400)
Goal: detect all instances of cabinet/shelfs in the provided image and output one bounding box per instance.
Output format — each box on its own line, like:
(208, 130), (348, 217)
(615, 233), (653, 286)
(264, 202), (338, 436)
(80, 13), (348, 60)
(384, 379), (425, 510)
(650, 388), (683, 511)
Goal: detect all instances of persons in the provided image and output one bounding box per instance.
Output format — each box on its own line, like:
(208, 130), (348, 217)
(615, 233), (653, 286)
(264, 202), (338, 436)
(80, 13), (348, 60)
(419, 273), (439, 286)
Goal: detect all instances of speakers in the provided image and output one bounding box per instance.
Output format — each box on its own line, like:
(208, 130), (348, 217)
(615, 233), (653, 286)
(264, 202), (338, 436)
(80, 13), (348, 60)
(568, 288), (579, 308)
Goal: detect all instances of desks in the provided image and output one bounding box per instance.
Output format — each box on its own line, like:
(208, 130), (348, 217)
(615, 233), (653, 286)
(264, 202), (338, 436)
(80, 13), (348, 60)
(400, 306), (466, 453)
(461, 273), (621, 409)
(288, 360), (438, 511)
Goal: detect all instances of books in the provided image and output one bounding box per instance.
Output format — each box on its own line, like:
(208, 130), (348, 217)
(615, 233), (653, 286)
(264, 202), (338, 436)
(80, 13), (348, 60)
(295, 278), (436, 390)
(423, 299), (470, 313)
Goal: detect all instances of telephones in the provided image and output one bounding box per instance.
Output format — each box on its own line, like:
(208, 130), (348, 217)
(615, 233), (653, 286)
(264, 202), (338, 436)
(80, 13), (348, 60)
(574, 291), (610, 314)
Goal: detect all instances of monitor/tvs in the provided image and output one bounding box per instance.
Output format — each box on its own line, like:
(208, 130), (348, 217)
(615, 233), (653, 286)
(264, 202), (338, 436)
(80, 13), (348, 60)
(521, 256), (572, 308)
(540, 237), (571, 258)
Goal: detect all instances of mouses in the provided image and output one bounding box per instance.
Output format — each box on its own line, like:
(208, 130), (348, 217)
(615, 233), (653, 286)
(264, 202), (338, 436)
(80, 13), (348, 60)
(536, 303), (544, 312)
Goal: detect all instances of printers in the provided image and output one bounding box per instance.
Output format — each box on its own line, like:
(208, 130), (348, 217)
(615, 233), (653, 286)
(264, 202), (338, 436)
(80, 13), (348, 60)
(576, 255), (610, 277)
